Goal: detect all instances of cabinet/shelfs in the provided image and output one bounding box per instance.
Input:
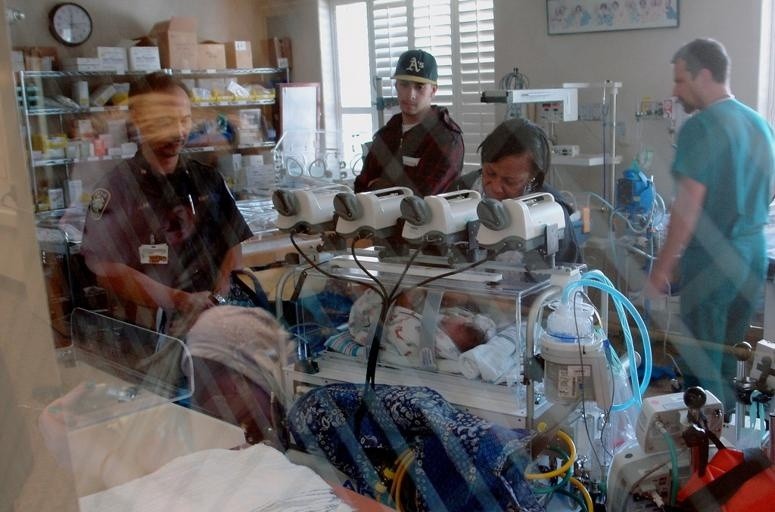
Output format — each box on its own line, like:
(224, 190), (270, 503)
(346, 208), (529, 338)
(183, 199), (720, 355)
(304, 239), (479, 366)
(18, 65), (281, 207)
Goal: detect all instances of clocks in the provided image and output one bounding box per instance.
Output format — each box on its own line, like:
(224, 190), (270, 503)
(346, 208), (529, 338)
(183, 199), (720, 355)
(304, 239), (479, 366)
(46, 3), (95, 47)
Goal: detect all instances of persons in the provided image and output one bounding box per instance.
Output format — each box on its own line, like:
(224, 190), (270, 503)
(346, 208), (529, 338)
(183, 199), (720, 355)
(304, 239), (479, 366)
(635, 41), (775, 422)
(352, 50), (463, 196)
(80, 70), (254, 384)
(431, 117), (580, 269)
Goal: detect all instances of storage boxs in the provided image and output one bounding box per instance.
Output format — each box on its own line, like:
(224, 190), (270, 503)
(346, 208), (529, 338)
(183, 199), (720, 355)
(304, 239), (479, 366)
(22, 15), (288, 76)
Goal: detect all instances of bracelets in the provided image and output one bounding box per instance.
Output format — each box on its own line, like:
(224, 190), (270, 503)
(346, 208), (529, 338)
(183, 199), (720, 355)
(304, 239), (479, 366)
(208, 293), (231, 307)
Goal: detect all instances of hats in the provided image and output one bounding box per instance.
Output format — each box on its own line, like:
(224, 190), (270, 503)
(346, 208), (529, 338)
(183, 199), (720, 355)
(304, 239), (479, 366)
(390, 49), (439, 87)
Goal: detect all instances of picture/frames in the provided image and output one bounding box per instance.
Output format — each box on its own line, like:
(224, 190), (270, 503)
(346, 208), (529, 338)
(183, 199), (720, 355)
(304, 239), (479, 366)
(276, 80), (320, 164)
(548, 0), (684, 37)
